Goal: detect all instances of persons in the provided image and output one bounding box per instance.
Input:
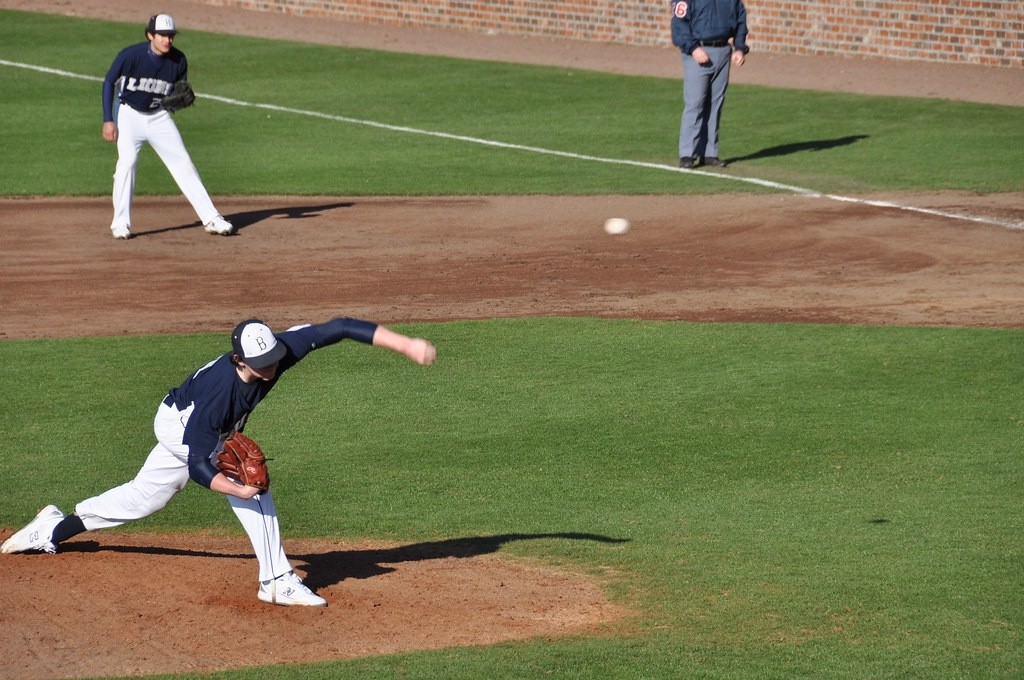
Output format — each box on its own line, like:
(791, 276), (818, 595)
(670, 0), (750, 169)
(0, 318), (439, 606)
(101, 13), (234, 239)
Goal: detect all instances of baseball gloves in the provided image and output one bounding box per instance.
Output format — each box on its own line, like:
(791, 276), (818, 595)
(161, 79), (196, 112)
(214, 432), (270, 491)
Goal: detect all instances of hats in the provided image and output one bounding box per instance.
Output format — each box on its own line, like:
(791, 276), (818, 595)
(232, 319), (286, 369)
(148, 14), (180, 34)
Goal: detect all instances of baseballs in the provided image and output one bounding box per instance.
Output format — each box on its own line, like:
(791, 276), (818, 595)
(604, 217), (630, 236)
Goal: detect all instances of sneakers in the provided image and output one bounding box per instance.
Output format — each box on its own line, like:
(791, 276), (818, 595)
(113, 226), (130, 239)
(204, 215), (233, 235)
(0, 504), (64, 554)
(257, 571), (328, 607)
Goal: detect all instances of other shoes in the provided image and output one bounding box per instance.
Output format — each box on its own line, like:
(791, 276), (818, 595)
(698, 156), (727, 167)
(679, 157), (694, 168)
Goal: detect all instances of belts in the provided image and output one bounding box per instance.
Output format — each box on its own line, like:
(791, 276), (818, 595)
(164, 396), (181, 411)
(698, 41), (729, 47)
